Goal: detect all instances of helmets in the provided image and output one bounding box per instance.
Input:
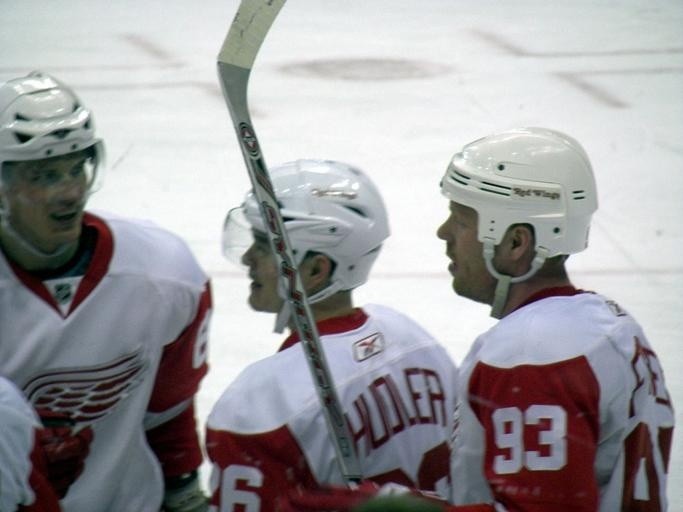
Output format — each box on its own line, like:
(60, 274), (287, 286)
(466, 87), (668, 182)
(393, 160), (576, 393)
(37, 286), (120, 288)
(0, 72), (105, 218)
(222, 158), (389, 292)
(440, 126), (598, 257)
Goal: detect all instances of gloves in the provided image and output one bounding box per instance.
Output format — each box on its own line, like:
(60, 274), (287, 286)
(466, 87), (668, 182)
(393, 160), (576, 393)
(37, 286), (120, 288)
(31, 425), (93, 497)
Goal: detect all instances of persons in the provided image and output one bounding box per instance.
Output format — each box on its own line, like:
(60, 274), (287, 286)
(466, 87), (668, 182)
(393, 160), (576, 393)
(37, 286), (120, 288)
(0, 376), (60, 512)
(1, 72), (213, 512)
(440, 126), (674, 512)
(202, 157), (455, 511)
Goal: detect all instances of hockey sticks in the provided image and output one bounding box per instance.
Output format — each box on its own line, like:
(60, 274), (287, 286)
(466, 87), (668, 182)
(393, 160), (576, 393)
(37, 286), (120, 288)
(217, 0), (363, 492)
